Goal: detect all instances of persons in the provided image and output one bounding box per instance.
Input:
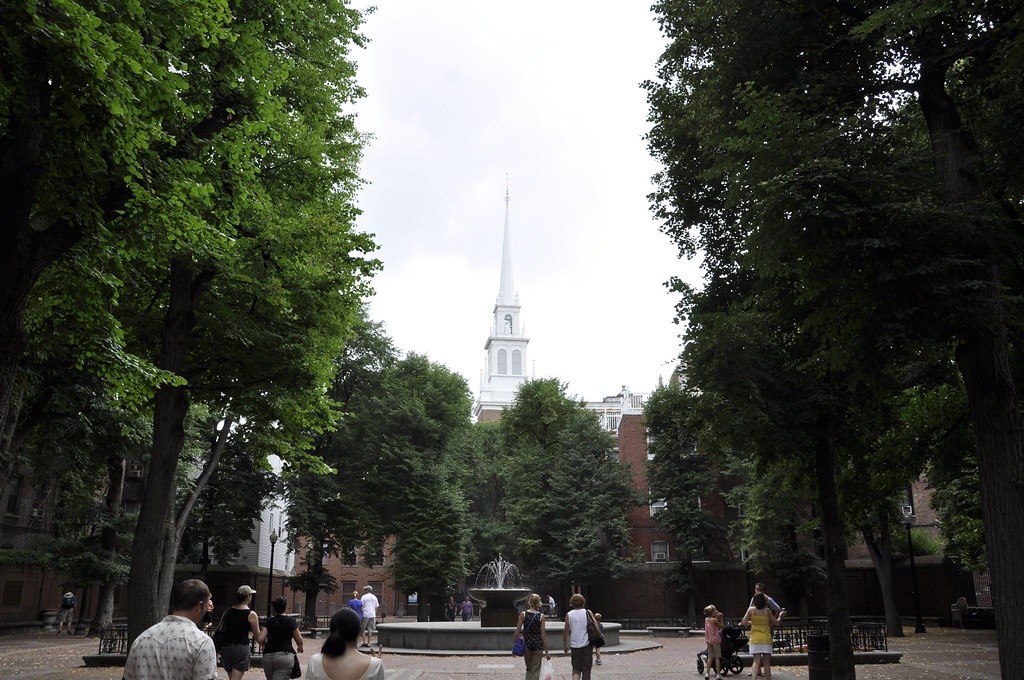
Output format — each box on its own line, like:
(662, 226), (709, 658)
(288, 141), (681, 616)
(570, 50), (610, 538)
(445, 596), (457, 622)
(306, 607), (385, 680)
(460, 595), (473, 621)
(546, 593), (556, 618)
(348, 591), (364, 621)
(512, 594), (550, 680)
(360, 585), (379, 647)
(738, 583), (786, 680)
(563, 593), (598, 680)
(57, 592), (77, 636)
(703, 605), (724, 680)
(203, 593), (214, 635)
(258, 596), (304, 680)
(122, 579), (219, 680)
(595, 613), (603, 665)
(219, 585), (261, 680)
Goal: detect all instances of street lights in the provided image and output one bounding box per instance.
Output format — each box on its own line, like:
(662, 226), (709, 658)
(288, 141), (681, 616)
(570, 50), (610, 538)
(900, 510), (927, 634)
(266, 528), (278, 620)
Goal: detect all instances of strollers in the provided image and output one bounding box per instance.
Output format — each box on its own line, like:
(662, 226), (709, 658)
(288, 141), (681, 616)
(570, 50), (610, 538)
(696, 624), (750, 677)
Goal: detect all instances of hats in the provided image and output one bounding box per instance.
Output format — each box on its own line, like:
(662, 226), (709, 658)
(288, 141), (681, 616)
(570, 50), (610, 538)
(237, 585), (257, 595)
(362, 585), (373, 591)
(64, 592), (73, 598)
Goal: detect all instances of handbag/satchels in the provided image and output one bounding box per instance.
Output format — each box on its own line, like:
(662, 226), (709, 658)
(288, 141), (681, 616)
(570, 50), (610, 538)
(513, 633), (525, 656)
(586, 608), (605, 648)
(211, 629), (225, 651)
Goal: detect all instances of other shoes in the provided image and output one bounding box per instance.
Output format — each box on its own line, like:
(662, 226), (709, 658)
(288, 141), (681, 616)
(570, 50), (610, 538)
(365, 644), (371, 647)
(748, 668), (761, 676)
(705, 673), (710, 680)
(761, 672), (772, 677)
(595, 658), (599, 662)
(597, 659), (602, 665)
(716, 674), (723, 680)
(361, 643), (366, 647)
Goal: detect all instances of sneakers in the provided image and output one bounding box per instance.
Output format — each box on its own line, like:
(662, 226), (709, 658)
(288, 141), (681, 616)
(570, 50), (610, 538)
(57, 631), (61, 635)
(67, 631), (72, 635)
